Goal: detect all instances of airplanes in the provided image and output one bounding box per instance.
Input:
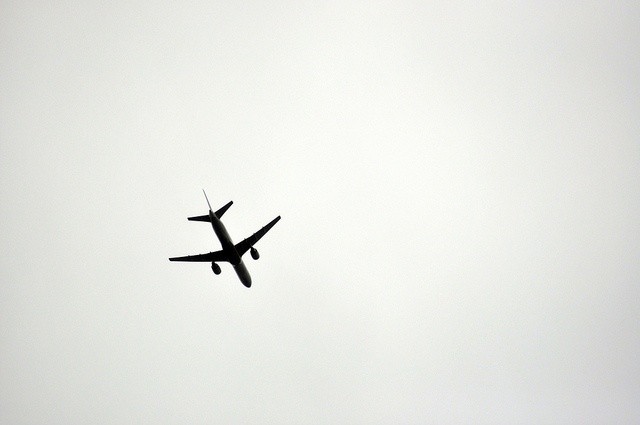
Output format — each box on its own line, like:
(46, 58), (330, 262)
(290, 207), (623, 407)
(169, 188), (281, 289)
